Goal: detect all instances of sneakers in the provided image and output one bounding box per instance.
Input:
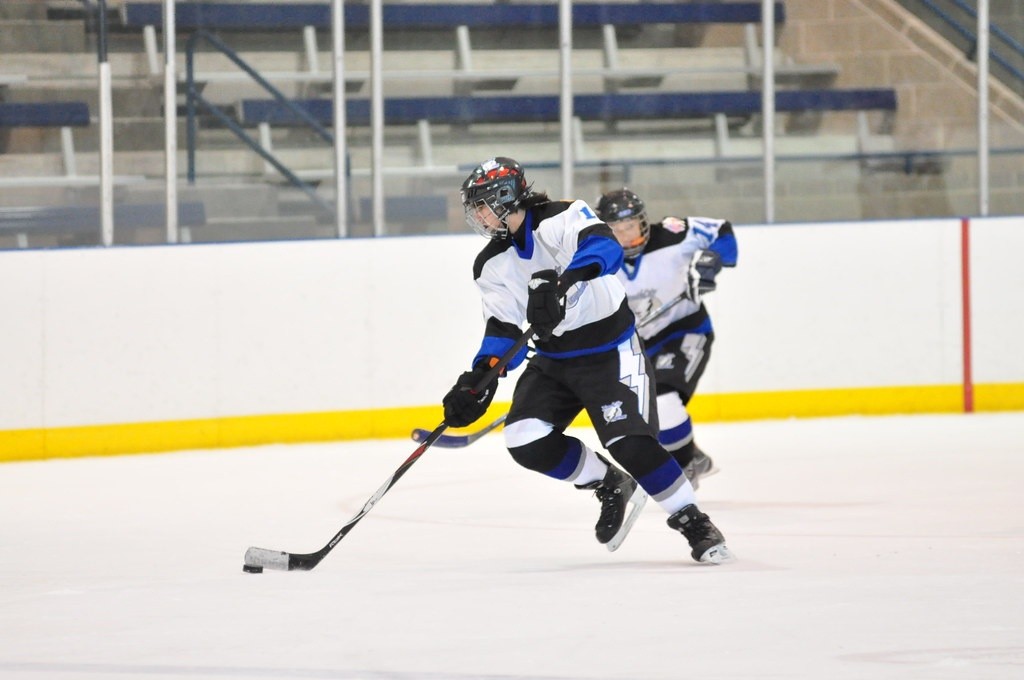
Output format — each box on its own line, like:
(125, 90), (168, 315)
(573, 450), (648, 553)
(683, 459), (701, 491)
(687, 444), (713, 477)
(666, 504), (730, 565)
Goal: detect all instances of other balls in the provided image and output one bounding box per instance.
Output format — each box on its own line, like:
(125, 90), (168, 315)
(242, 567), (263, 574)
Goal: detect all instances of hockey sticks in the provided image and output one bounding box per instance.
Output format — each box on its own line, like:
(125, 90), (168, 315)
(245, 324), (537, 570)
(411, 291), (691, 447)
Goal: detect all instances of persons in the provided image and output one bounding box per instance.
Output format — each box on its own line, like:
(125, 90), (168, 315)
(594, 186), (738, 490)
(442, 157), (729, 563)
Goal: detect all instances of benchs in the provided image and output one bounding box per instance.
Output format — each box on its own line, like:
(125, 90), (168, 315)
(0, 0), (899, 252)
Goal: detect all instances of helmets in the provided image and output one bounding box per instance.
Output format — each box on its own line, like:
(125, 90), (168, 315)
(460, 157), (528, 242)
(594, 188), (649, 257)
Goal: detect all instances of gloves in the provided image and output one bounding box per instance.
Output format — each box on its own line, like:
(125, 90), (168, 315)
(682, 249), (720, 304)
(442, 364), (499, 429)
(527, 271), (567, 339)
